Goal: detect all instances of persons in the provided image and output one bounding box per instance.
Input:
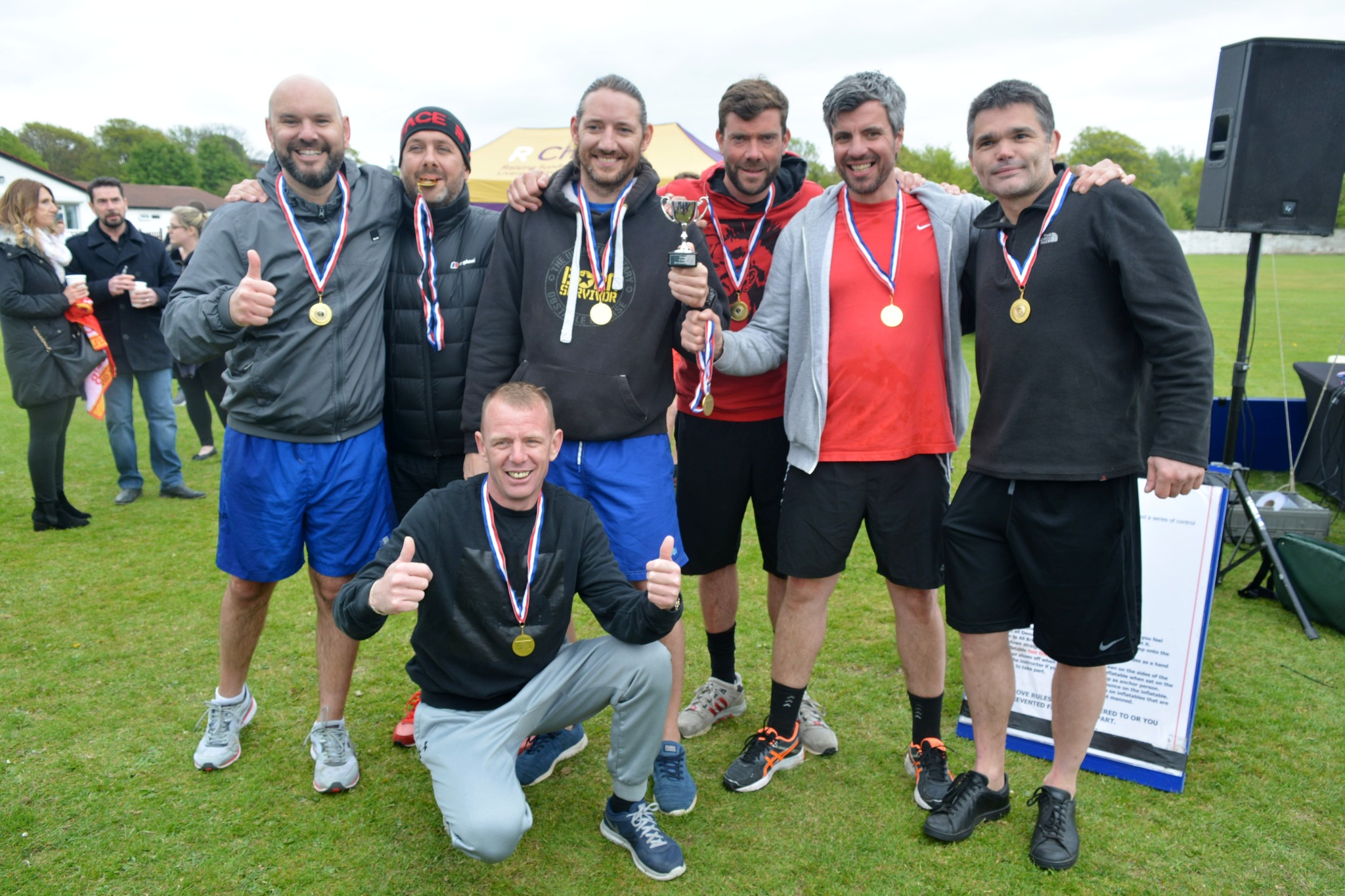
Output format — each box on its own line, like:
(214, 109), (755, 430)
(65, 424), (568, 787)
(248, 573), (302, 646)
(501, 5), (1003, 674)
(680, 74), (1135, 813)
(159, 81), (401, 793)
(531, 79), (969, 759)
(224, 107), (503, 757)
(0, 180), (231, 534)
(458, 74), (730, 819)
(922, 81), (1207, 869)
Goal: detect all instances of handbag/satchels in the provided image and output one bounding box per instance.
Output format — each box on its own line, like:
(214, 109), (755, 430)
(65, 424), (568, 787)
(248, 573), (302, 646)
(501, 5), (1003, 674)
(48, 331), (107, 387)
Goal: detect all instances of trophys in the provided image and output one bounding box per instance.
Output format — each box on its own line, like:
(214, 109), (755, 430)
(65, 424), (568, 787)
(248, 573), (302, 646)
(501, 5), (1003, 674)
(659, 194), (710, 267)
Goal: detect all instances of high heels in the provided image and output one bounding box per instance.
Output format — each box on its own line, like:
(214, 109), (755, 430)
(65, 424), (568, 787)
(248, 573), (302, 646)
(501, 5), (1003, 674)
(32, 497), (89, 531)
(58, 491), (93, 521)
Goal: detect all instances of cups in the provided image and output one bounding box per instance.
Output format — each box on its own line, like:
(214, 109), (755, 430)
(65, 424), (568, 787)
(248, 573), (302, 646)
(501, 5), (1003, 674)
(65, 274), (87, 289)
(128, 281), (147, 307)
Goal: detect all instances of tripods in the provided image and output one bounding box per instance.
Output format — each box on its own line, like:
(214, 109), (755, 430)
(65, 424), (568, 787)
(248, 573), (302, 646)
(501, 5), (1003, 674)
(1214, 231), (1317, 641)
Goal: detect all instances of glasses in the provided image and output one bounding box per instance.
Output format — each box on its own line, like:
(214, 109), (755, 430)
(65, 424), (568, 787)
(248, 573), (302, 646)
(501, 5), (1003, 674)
(168, 225), (185, 232)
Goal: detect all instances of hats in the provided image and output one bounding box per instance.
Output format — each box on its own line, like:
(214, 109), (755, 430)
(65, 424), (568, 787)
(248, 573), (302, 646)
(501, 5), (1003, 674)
(398, 106), (472, 173)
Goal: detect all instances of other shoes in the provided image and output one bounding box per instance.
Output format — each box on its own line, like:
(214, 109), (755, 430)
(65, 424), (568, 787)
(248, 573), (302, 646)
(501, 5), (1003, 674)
(193, 446), (219, 461)
(114, 487), (140, 505)
(159, 482), (206, 499)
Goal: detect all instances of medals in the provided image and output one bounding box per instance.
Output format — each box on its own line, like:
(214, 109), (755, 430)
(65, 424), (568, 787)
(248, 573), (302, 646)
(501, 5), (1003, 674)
(512, 634), (535, 657)
(730, 301), (749, 321)
(881, 305), (903, 327)
(309, 295), (333, 326)
(702, 395), (715, 415)
(415, 177), (440, 188)
(1010, 298), (1031, 323)
(589, 303), (612, 325)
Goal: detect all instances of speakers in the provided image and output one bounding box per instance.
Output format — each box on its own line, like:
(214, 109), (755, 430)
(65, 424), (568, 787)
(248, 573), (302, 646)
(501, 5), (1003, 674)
(1195, 37), (1345, 237)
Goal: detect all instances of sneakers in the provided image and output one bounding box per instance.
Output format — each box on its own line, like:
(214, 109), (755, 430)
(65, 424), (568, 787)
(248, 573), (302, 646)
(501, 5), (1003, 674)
(515, 720), (589, 788)
(676, 671), (747, 738)
(797, 693), (838, 756)
(650, 739), (697, 818)
(302, 706), (359, 794)
(393, 692), (425, 750)
(1027, 784), (1080, 872)
(905, 732), (958, 811)
(599, 794), (686, 882)
(194, 681), (258, 770)
(723, 716), (805, 793)
(923, 768), (1011, 842)
